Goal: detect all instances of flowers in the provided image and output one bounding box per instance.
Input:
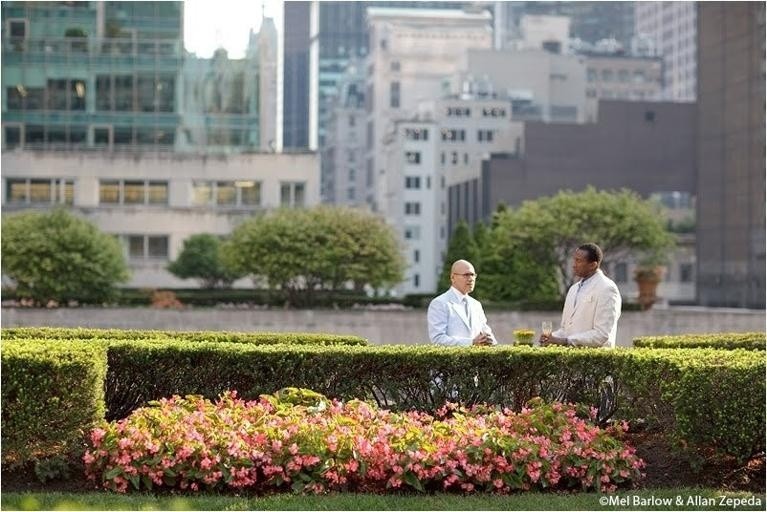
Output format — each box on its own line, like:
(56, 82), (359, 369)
(513, 328), (536, 343)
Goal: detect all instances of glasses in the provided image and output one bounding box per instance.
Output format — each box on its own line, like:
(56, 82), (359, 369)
(455, 273), (477, 279)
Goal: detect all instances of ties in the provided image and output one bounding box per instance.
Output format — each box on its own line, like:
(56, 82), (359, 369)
(463, 298), (469, 322)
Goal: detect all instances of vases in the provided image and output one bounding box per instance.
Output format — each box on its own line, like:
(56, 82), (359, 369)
(513, 341), (535, 347)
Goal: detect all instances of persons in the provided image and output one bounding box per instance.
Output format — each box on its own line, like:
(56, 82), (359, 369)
(429, 258), (496, 397)
(539, 244), (621, 416)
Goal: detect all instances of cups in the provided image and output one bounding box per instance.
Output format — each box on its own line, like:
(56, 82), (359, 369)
(541, 319), (553, 338)
